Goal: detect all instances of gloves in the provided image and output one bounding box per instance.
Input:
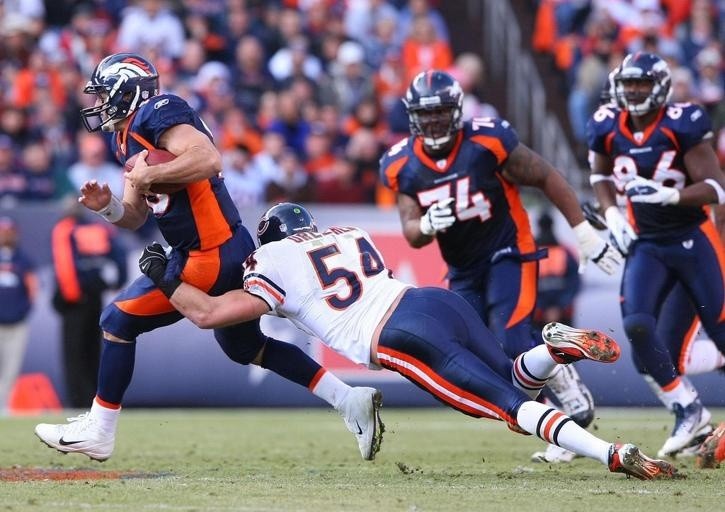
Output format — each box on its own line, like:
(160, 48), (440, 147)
(624, 175), (680, 207)
(605, 206), (639, 254)
(139, 241), (183, 299)
(573, 220), (622, 276)
(420, 198), (456, 236)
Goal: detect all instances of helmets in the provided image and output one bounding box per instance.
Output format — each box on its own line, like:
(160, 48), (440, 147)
(80, 52), (159, 132)
(600, 52), (672, 117)
(257, 203), (318, 248)
(401, 68), (464, 150)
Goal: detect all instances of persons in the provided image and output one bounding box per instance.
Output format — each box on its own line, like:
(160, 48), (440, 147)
(1, 0), (724, 202)
(49, 203), (128, 409)
(139, 200), (678, 480)
(34, 51), (385, 461)
(0, 212), (40, 413)
(375, 67), (626, 468)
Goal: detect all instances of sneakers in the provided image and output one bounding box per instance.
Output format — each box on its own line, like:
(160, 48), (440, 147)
(658, 396), (715, 460)
(608, 443), (687, 480)
(696, 422), (725, 468)
(344, 387), (386, 461)
(531, 444), (585, 464)
(542, 322), (621, 364)
(35, 412), (115, 462)
(546, 363), (594, 428)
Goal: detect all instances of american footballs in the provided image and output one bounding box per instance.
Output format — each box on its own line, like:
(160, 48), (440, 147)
(124, 150), (186, 194)
